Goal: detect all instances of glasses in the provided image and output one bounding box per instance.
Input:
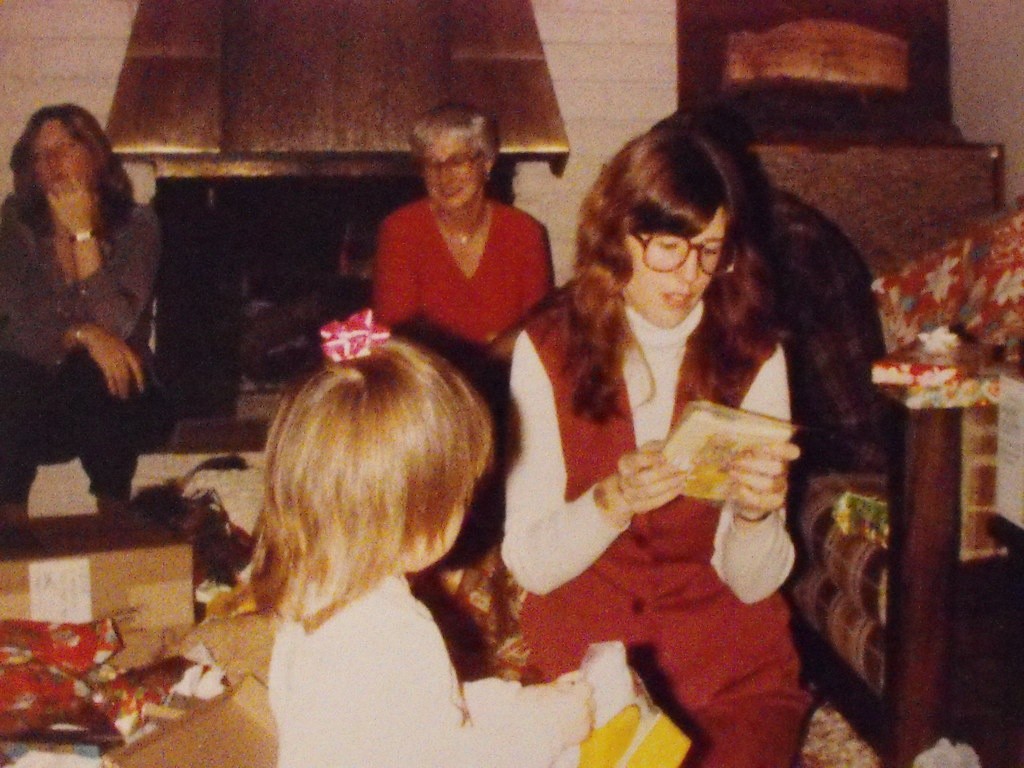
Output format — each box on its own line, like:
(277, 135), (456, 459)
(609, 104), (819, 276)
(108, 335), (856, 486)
(627, 229), (739, 278)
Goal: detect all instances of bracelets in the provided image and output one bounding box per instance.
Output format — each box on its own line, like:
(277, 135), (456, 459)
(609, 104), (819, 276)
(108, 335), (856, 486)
(74, 321), (88, 347)
(69, 230), (96, 243)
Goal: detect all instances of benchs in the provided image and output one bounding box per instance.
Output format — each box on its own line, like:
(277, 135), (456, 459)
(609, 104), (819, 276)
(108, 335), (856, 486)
(784, 196), (1024, 768)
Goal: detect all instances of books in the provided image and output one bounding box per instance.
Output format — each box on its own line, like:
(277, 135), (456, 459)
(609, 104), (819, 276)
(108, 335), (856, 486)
(657, 401), (796, 505)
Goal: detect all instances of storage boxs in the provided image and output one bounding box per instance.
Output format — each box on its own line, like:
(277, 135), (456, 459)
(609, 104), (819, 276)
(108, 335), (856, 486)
(0, 491), (198, 627)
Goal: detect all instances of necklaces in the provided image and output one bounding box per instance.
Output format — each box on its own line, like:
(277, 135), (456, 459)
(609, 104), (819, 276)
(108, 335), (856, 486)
(459, 233), (472, 247)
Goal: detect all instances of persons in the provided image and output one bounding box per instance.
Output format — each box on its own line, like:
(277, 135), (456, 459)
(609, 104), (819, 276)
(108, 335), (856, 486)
(368, 105), (553, 396)
(248, 311), (597, 768)
(0, 104), (163, 532)
(501, 133), (810, 768)
